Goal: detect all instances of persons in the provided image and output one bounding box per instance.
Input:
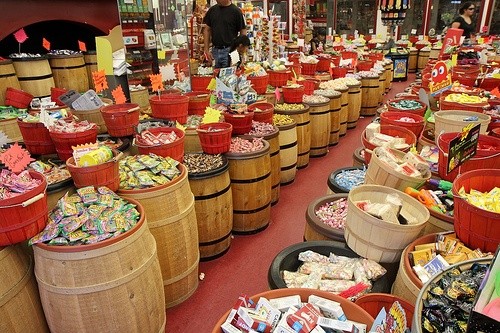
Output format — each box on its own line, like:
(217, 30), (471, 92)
(204, 0), (247, 78)
(309, 28), (326, 54)
(452, 3), (476, 38)
(437, 9), (445, 31)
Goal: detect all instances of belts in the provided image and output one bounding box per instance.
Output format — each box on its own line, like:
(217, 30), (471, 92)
(215, 45), (231, 49)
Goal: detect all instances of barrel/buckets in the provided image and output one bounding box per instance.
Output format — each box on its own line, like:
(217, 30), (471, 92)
(306, 80), (363, 158)
(439, 86), (500, 138)
(376, 69), (441, 117)
(185, 101), (274, 154)
(408, 49), (441, 72)
(391, 206), (494, 333)
(48, 51), (97, 106)
(330, 42), (383, 79)
(360, 111), (438, 193)
(0, 117), (23, 143)
(183, 135), (271, 263)
(97, 103), (185, 164)
(268, 146), (430, 303)
(248, 45), (333, 104)
(211, 288), (415, 333)
(360, 59), (394, 117)
(130, 75), (215, 124)
(224, 103), (311, 206)
(450, 55), (500, 100)
(18, 98), (113, 161)
(396, 36), (439, 49)
(434, 110), (500, 253)
(0, 55), (70, 108)
(0, 161), (199, 333)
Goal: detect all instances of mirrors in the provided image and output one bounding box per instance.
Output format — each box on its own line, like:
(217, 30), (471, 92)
(117, 0), (192, 95)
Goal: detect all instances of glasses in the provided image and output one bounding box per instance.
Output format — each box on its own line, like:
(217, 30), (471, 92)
(468, 8), (475, 10)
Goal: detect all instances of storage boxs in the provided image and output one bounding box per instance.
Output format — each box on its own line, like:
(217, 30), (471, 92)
(220, 293), (366, 333)
